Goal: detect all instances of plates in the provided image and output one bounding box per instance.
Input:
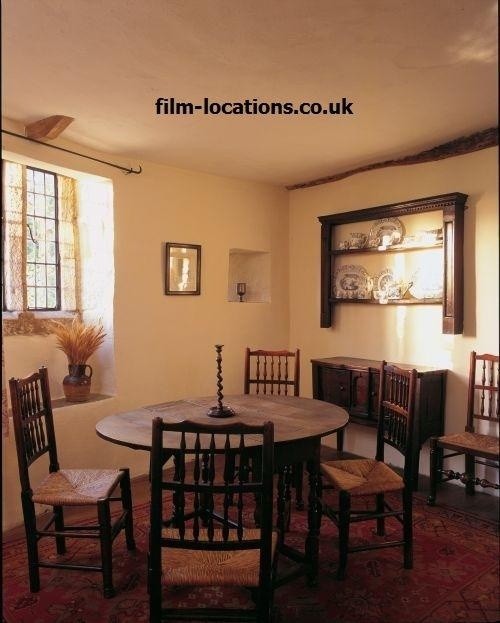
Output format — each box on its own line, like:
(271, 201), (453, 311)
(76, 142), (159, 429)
(373, 267), (396, 290)
(369, 216), (404, 245)
(331, 263), (368, 298)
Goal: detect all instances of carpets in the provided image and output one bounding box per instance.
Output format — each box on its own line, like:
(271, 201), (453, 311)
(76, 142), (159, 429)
(1, 479), (500, 620)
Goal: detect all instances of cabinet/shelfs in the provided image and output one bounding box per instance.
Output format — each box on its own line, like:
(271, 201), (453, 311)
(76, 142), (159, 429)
(309, 356), (450, 489)
(316, 189), (468, 335)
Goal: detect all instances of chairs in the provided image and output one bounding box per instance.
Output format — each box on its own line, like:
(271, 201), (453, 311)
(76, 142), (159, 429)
(242, 345), (301, 504)
(6, 366), (138, 601)
(148, 414), (282, 621)
(428, 350), (499, 508)
(311, 359), (420, 575)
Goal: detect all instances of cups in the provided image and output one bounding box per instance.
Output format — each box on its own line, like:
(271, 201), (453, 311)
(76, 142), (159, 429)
(337, 240), (348, 249)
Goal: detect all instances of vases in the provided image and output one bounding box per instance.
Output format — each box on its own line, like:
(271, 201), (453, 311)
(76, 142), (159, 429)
(62, 363), (94, 404)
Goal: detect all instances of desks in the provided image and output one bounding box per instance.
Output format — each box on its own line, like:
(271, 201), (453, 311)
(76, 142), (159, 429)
(96, 393), (350, 590)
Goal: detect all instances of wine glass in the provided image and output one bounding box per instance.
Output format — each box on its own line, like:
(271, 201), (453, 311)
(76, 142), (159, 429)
(236, 282), (246, 302)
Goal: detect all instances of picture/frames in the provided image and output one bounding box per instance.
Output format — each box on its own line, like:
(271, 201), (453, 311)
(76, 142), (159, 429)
(164, 242), (201, 295)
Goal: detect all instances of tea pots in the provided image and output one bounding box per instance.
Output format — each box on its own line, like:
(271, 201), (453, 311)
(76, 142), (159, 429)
(349, 230), (368, 248)
